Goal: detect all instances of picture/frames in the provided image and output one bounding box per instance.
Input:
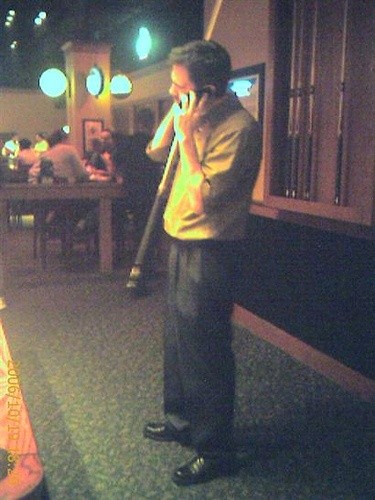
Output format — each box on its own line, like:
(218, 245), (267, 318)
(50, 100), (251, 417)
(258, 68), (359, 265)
(229, 62), (266, 133)
(82, 118), (104, 156)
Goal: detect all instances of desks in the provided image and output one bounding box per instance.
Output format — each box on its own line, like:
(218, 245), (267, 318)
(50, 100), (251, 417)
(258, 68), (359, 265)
(0, 182), (128, 281)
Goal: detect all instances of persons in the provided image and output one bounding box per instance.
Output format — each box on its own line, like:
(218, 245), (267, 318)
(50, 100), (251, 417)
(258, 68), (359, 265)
(144, 39), (262, 487)
(0, 108), (167, 273)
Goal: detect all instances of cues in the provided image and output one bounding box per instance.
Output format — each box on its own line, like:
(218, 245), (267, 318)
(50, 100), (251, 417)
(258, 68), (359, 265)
(333, 1), (349, 206)
(291, 1), (304, 198)
(284, 0), (296, 197)
(127, 0), (224, 292)
(303, 0), (318, 201)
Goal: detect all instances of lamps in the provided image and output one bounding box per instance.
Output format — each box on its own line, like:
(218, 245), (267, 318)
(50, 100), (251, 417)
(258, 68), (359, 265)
(84, 64), (105, 99)
(110, 73), (134, 99)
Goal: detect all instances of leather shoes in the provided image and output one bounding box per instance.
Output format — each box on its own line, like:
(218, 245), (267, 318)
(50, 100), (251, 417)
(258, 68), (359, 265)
(172, 450), (242, 487)
(143, 411), (196, 442)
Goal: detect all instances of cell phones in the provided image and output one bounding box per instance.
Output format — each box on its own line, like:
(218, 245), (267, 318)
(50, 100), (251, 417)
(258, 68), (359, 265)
(179, 87), (211, 109)
